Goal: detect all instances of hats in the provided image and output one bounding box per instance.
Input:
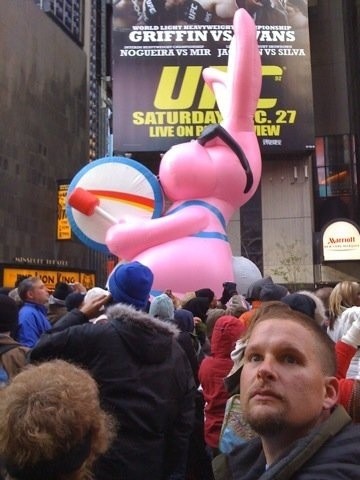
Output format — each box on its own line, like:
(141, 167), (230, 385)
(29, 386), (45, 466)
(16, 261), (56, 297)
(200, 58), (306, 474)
(108, 260), (153, 308)
(245, 276), (273, 303)
(195, 288), (214, 303)
(184, 297), (209, 322)
(259, 284), (288, 304)
(280, 294), (317, 321)
(65, 293), (85, 311)
(175, 309), (195, 332)
(149, 294), (174, 321)
(53, 282), (71, 301)
(0, 295), (20, 333)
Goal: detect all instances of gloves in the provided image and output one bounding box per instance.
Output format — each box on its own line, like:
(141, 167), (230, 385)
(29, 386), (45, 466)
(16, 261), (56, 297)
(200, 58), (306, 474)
(340, 306), (360, 349)
(222, 282), (238, 297)
(218, 289), (232, 304)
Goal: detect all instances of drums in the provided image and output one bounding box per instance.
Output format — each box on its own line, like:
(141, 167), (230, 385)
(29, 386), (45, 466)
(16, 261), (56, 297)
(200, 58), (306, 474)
(66, 156), (166, 254)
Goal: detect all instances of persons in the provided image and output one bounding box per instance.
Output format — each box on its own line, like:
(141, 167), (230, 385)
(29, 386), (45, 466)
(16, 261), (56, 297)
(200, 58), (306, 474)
(0, 260), (360, 480)
(113, 0), (309, 49)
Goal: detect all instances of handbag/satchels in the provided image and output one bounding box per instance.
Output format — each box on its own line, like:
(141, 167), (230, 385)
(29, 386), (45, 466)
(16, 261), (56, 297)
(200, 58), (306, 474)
(219, 394), (258, 455)
